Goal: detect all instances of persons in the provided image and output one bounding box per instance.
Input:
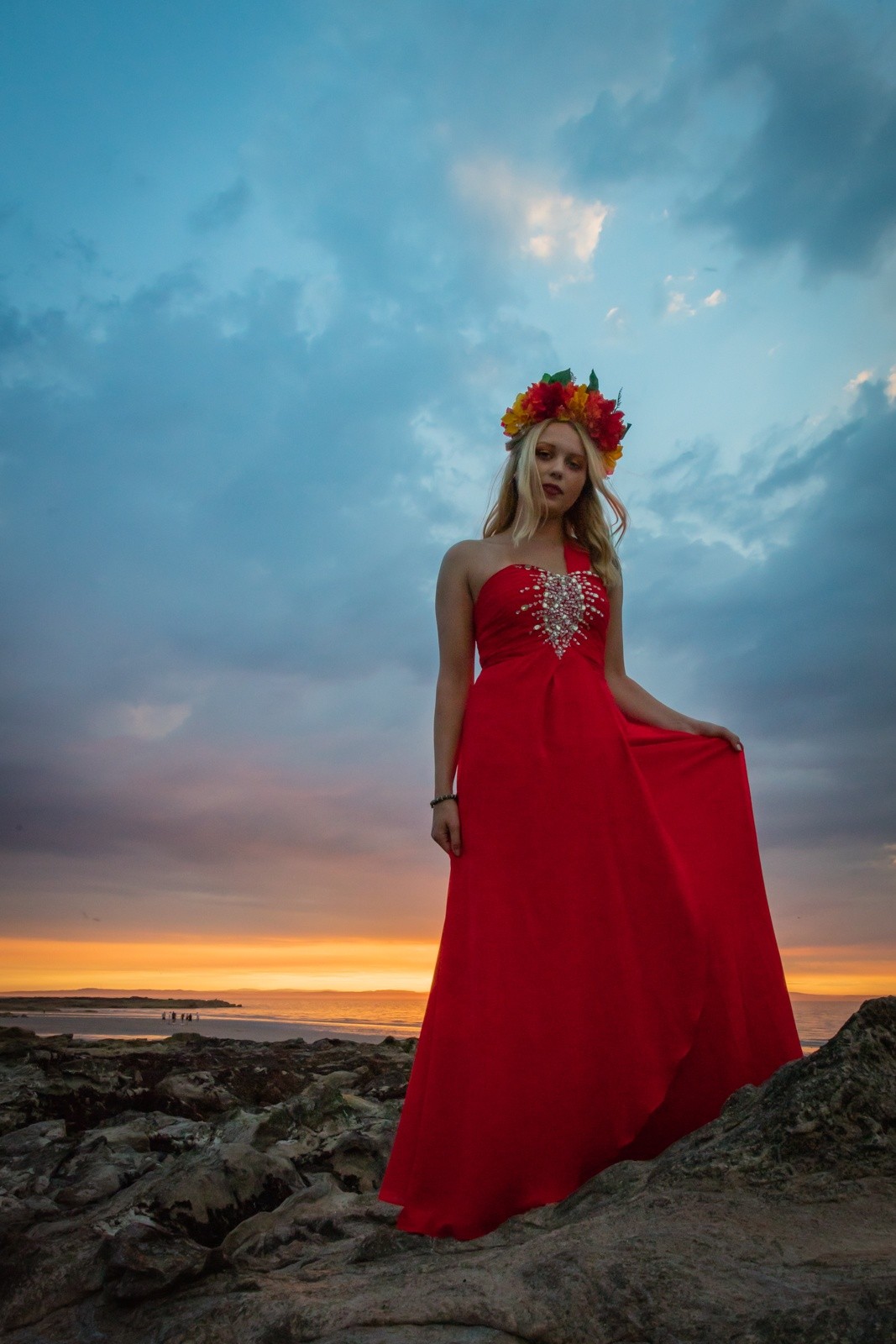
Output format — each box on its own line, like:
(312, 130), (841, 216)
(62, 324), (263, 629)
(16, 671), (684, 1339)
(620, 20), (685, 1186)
(371, 365), (807, 1239)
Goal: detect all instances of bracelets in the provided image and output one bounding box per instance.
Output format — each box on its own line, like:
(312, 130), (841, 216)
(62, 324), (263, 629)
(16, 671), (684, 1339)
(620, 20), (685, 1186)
(429, 793), (458, 810)
